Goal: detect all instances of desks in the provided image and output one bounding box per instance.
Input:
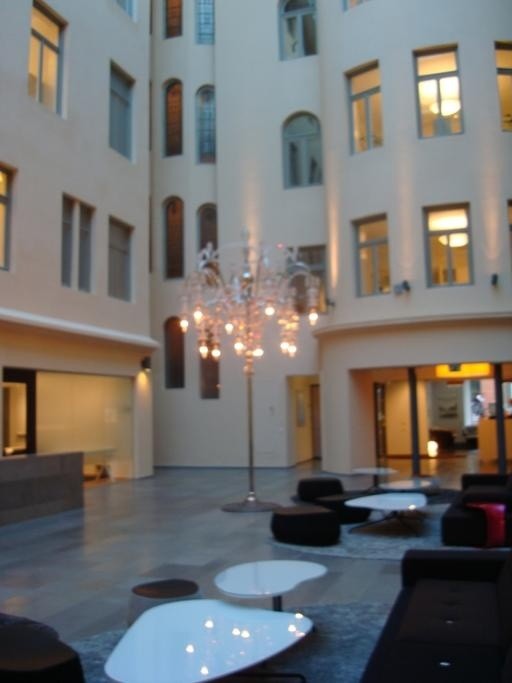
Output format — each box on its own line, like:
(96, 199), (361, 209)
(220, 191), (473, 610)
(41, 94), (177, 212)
(98, 598), (316, 682)
(211, 556), (331, 613)
(340, 489), (431, 533)
(350, 467), (398, 494)
(379, 476), (433, 495)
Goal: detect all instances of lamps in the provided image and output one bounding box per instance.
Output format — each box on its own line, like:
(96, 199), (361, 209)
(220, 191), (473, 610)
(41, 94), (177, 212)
(391, 280), (411, 295)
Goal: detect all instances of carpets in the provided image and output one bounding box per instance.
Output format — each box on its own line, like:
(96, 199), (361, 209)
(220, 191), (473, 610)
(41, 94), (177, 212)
(62, 598), (390, 683)
(261, 501), (511, 558)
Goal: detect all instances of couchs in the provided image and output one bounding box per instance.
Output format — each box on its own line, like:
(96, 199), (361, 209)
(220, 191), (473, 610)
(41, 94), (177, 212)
(356, 541), (512, 681)
(439, 471), (512, 546)
(0, 608), (86, 681)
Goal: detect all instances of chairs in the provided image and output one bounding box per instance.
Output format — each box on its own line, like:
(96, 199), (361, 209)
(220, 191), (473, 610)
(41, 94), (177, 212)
(291, 474), (374, 524)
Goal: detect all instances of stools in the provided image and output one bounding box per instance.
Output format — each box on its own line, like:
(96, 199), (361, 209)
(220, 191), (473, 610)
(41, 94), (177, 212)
(128, 574), (201, 625)
(268, 502), (342, 547)
